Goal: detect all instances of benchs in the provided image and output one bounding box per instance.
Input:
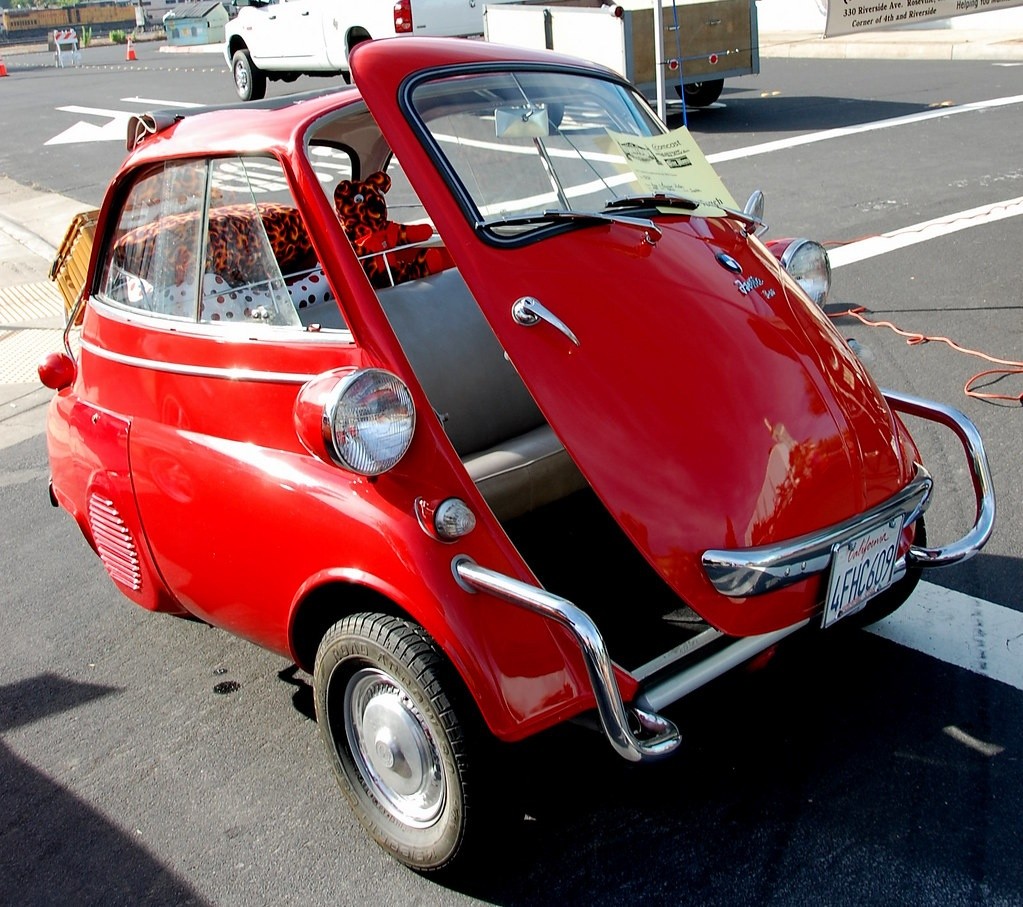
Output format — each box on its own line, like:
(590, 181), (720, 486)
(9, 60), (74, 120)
(268, 264), (590, 523)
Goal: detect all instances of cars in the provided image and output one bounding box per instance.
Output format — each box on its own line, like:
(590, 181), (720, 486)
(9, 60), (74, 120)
(37, 35), (998, 874)
(223, 0), (525, 102)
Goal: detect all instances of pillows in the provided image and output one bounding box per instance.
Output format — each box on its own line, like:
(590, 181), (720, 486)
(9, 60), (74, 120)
(112, 202), (313, 289)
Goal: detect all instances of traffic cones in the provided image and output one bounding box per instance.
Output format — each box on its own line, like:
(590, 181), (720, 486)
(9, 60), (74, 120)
(126, 35), (138, 61)
(0, 56), (10, 78)
